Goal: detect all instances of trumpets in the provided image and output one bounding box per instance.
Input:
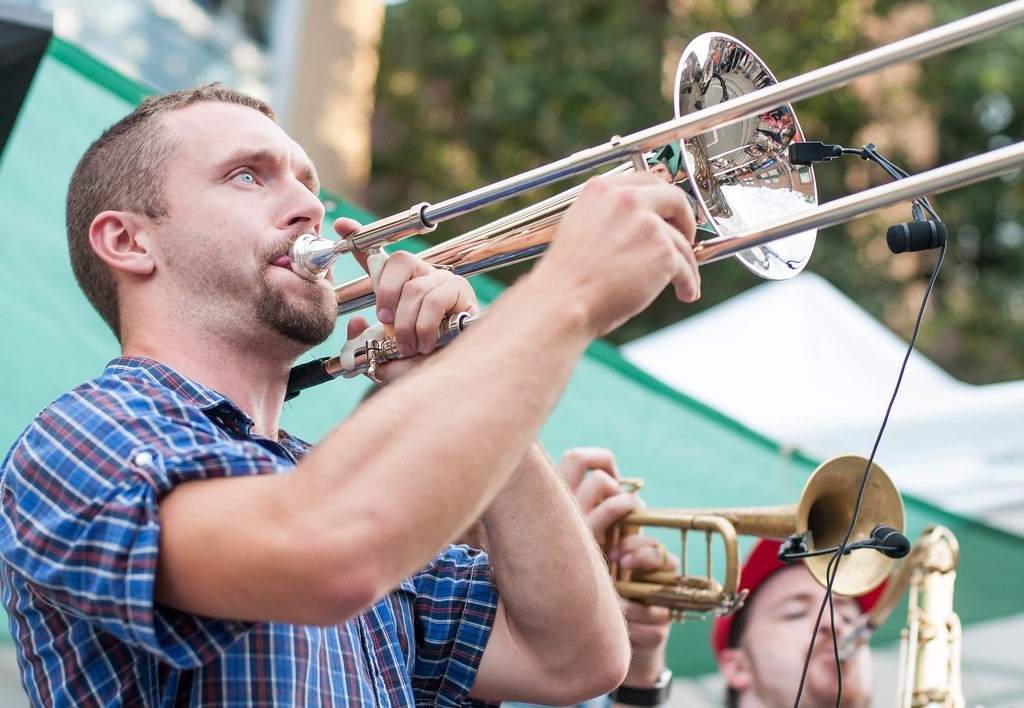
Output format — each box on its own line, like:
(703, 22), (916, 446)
(609, 454), (907, 625)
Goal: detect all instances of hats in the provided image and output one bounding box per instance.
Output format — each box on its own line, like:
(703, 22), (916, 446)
(711, 539), (891, 656)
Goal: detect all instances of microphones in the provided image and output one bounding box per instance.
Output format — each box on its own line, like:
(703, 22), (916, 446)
(870, 525), (911, 560)
(887, 220), (947, 254)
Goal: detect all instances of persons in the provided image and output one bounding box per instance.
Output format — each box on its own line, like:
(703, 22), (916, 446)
(712, 535), (892, 708)
(0, 86), (702, 707)
(359, 383), (674, 707)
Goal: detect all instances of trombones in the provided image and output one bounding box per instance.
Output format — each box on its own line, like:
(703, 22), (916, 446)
(282, 0), (1024, 403)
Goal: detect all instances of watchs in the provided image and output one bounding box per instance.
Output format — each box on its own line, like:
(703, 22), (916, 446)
(607, 666), (674, 707)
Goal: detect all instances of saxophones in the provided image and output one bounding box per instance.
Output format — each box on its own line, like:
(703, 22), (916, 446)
(834, 522), (967, 708)
